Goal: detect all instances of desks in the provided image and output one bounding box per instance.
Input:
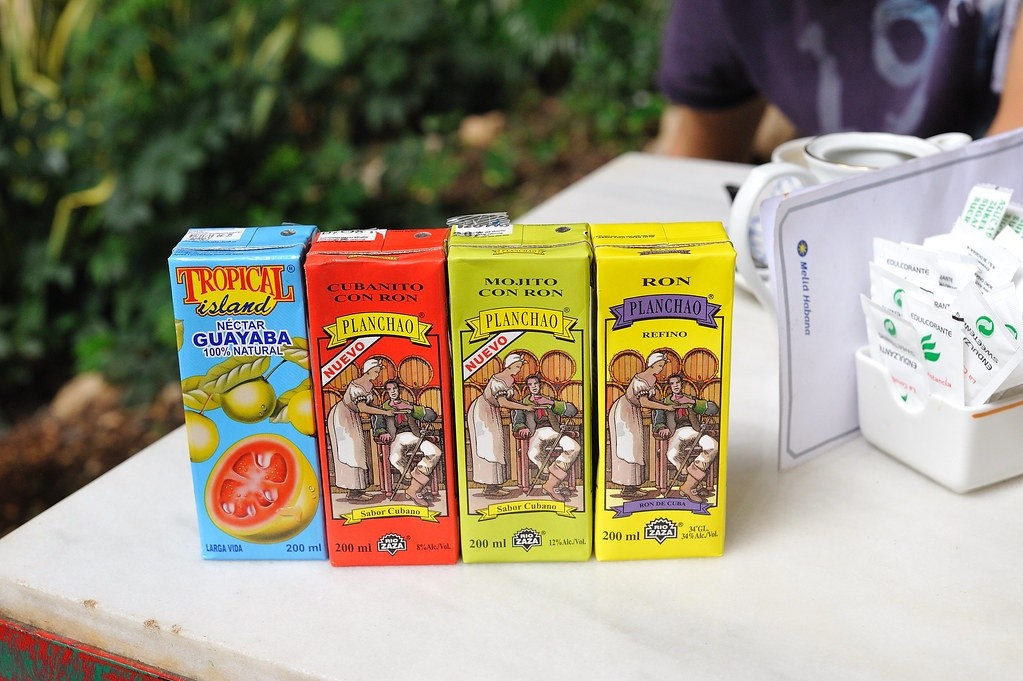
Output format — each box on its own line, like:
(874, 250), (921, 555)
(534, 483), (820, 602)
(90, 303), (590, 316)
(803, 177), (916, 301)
(0, 149), (1022, 680)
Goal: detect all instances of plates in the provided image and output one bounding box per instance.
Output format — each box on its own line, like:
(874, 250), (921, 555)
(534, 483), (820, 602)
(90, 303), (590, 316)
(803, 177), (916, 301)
(733, 272), (767, 299)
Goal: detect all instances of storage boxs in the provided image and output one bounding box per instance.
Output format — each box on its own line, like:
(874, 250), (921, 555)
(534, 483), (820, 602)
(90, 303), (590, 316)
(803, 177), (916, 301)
(854, 348), (1020, 494)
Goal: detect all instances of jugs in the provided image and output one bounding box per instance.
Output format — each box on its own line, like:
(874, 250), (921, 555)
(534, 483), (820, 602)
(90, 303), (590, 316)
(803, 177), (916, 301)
(728, 131), (973, 322)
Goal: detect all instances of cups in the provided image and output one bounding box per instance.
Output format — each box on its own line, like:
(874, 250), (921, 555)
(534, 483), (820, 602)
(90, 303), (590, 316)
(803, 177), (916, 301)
(748, 136), (816, 268)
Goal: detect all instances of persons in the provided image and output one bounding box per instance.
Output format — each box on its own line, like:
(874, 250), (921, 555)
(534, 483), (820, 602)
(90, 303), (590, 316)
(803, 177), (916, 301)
(643, 0), (1023, 167)
